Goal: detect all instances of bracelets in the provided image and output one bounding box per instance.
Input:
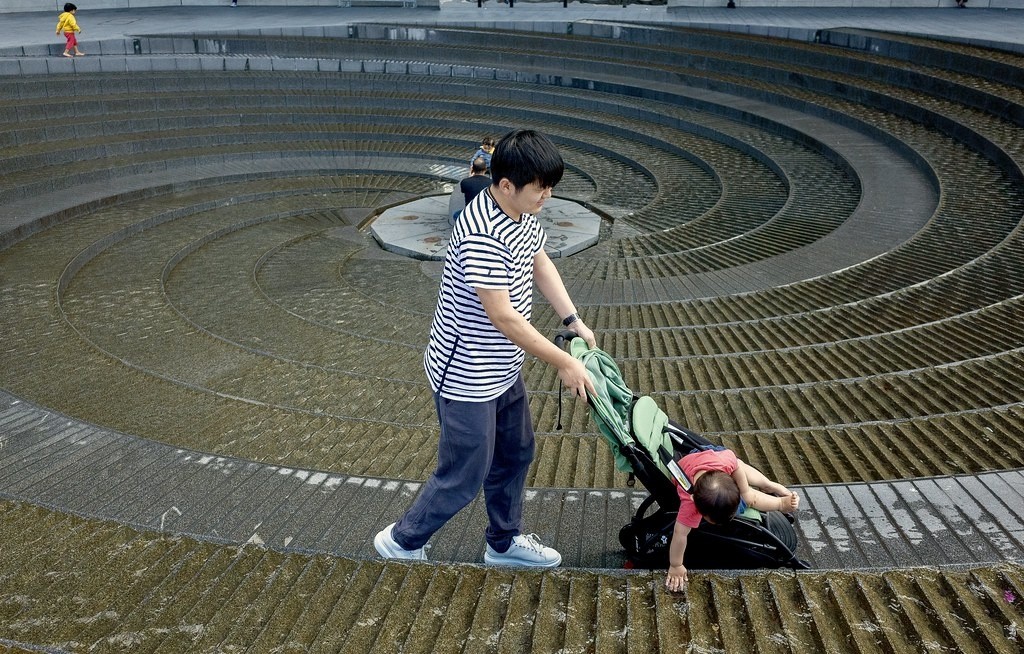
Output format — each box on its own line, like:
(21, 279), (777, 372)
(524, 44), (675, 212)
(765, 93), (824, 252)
(562, 313), (580, 327)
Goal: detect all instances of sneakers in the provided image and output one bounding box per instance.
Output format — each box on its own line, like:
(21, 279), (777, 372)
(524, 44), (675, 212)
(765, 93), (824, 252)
(483, 535), (562, 568)
(374, 523), (427, 561)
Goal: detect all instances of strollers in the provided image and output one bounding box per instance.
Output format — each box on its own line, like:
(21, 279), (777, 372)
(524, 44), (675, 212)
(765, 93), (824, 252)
(554, 330), (811, 570)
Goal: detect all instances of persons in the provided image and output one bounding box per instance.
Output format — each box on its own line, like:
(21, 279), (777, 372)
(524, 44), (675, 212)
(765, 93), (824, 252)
(666, 445), (800, 593)
(55, 3), (85, 57)
(374, 130), (598, 568)
(452, 137), (495, 222)
